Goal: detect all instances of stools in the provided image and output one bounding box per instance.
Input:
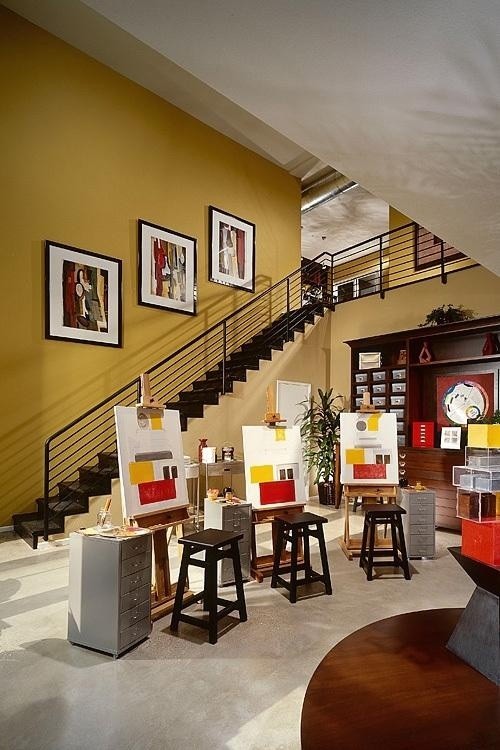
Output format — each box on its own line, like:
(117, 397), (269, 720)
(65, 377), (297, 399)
(271, 512), (332, 603)
(170, 528), (247, 645)
(358, 504), (411, 581)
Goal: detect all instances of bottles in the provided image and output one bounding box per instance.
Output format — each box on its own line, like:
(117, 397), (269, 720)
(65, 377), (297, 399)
(96, 506), (112, 528)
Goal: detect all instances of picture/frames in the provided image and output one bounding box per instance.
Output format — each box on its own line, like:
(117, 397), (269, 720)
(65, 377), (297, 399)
(137, 218), (197, 317)
(440, 426), (462, 451)
(208, 204), (256, 294)
(413, 220), (471, 274)
(43, 239), (122, 349)
(431, 368), (498, 436)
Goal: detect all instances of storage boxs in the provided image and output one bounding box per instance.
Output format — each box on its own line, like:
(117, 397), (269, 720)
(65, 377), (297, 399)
(411, 421), (434, 449)
(354, 369), (406, 414)
(359, 352), (382, 370)
(390, 409), (407, 447)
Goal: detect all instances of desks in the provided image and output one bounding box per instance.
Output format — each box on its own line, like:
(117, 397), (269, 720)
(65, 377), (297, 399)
(194, 458), (244, 532)
(182, 462), (199, 532)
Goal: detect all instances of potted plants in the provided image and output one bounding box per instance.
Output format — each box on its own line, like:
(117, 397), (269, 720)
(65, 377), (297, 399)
(293, 387), (346, 506)
(467, 410), (500, 450)
(418, 304), (478, 326)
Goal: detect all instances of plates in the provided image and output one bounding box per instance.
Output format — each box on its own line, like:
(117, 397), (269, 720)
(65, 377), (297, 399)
(100, 527), (148, 537)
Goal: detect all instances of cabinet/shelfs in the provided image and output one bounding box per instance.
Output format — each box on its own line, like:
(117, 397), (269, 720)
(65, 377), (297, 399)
(67, 525), (154, 661)
(301, 256), (330, 307)
(343, 313), (500, 533)
(450, 446), (500, 568)
(204, 496), (252, 589)
(396, 487), (436, 559)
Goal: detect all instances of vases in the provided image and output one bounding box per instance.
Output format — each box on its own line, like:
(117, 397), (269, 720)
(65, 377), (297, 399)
(198, 439), (208, 462)
(482, 332), (500, 356)
(418, 342), (433, 364)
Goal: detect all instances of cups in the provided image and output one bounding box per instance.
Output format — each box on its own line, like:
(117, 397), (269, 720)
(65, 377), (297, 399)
(225, 492), (232, 503)
